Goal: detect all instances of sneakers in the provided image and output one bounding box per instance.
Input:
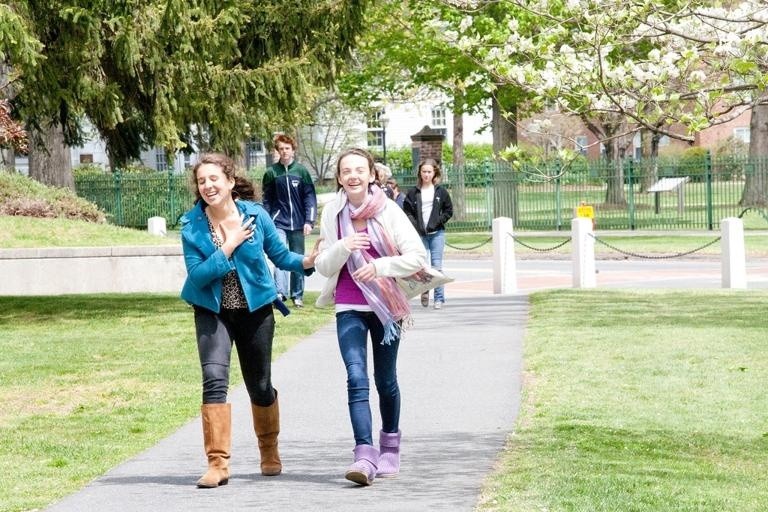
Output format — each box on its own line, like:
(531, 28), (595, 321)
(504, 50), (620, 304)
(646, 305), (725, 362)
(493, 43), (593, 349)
(420, 290), (430, 308)
(293, 299), (304, 308)
(434, 301), (443, 310)
(277, 293), (283, 302)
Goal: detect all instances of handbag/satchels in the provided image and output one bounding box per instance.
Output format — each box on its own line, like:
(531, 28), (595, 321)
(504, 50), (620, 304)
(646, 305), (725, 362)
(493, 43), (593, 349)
(395, 266), (454, 301)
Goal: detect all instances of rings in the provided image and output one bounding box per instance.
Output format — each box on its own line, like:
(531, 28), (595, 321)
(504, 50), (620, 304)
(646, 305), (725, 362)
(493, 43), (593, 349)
(248, 226), (254, 233)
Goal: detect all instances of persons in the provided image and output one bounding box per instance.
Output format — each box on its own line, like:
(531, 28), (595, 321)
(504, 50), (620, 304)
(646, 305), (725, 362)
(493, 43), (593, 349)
(260, 135), (318, 309)
(402, 159), (454, 311)
(384, 175), (407, 209)
(316, 146), (456, 487)
(178, 151), (326, 488)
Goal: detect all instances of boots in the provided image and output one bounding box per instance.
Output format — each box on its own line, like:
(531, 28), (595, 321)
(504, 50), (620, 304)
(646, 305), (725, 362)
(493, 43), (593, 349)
(194, 401), (232, 488)
(251, 385), (283, 476)
(344, 444), (380, 485)
(376, 427), (402, 478)
